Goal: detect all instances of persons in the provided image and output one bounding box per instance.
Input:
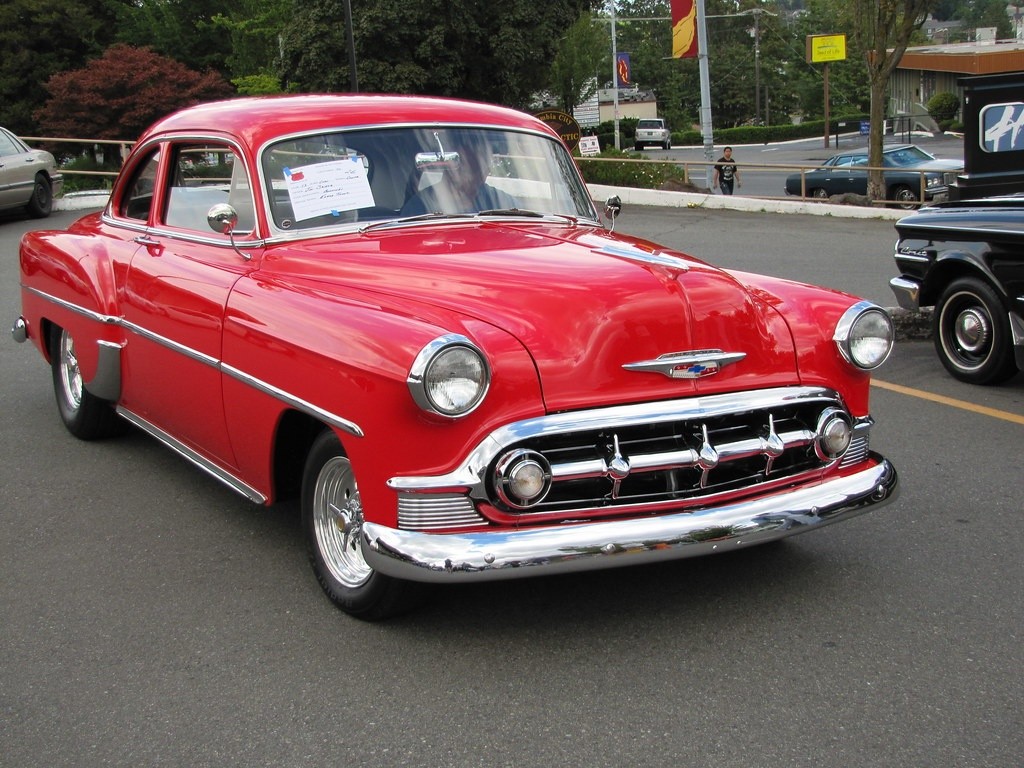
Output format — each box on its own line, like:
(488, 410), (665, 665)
(713, 146), (741, 195)
(399, 130), (516, 216)
